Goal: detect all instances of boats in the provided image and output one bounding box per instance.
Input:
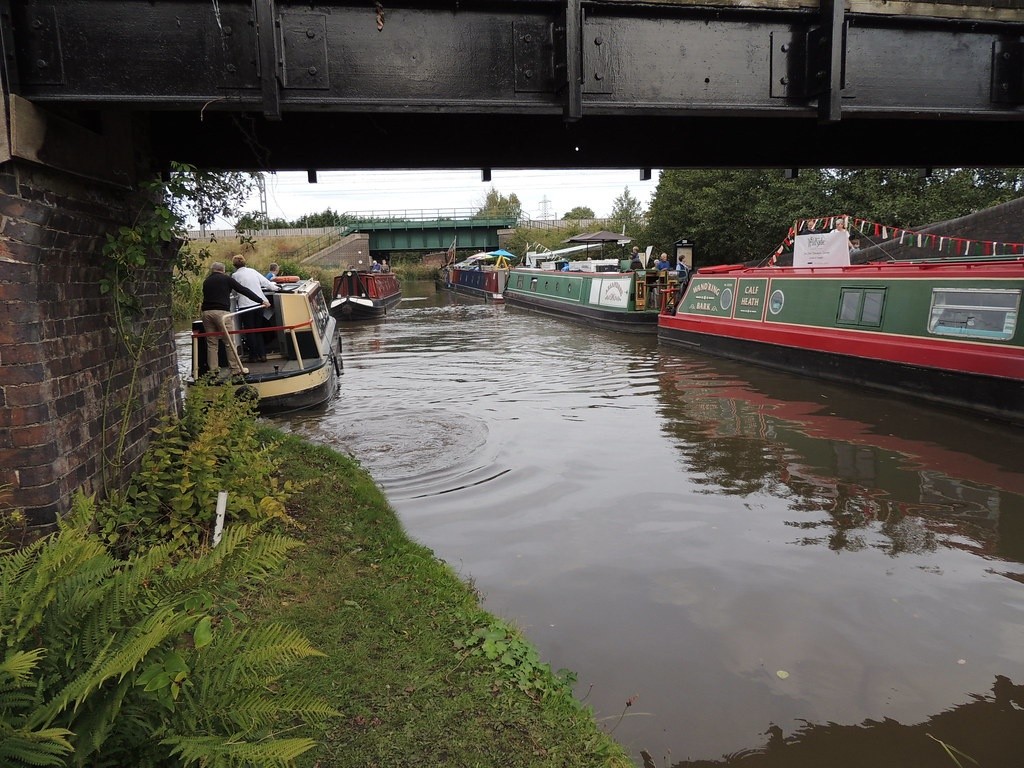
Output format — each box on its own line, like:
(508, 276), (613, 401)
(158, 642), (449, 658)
(183, 278), (344, 418)
(435, 253), (513, 300)
(329, 269), (403, 318)
(657, 216), (1024, 429)
(502, 229), (683, 334)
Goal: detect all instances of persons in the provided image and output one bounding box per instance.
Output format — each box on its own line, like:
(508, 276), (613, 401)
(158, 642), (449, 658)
(652, 260), (660, 269)
(373, 260), (389, 272)
(630, 247), (640, 261)
(830, 219), (855, 252)
(802, 222), (821, 234)
(232, 255), (282, 363)
(658, 253), (670, 270)
(266, 263), (279, 280)
(201, 262), (271, 376)
(675, 254), (689, 299)
(850, 239), (860, 252)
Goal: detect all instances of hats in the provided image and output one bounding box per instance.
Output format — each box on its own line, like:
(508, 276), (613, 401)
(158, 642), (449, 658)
(373, 260), (377, 263)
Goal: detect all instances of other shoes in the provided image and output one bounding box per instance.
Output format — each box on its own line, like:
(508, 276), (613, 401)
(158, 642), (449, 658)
(245, 357), (258, 363)
(261, 355), (267, 362)
(209, 369), (220, 379)
(232, 367), (250, 376)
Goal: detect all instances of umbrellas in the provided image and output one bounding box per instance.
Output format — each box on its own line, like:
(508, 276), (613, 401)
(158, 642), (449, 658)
(561, 231), (638, 258)
(468, 249), (517, 259)
